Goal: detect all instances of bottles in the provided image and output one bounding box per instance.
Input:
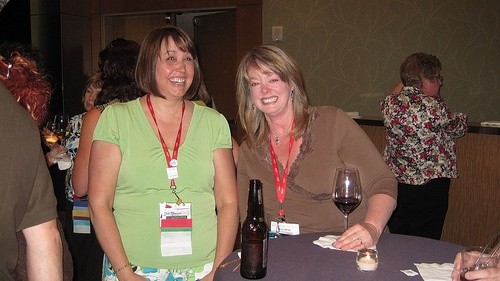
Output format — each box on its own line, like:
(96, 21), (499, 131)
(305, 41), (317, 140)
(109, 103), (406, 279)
(240, 180), (269, 279)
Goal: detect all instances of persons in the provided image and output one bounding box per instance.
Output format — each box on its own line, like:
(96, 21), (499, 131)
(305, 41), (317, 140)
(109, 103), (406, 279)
(0, 25), (240, 281)
(379, 53), (470, 241)
(235, 45), (399, 252)
(451, 251), (500, 281)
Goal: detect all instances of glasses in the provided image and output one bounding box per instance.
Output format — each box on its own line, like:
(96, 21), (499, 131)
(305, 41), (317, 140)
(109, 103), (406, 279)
(429, 75), (443, 81)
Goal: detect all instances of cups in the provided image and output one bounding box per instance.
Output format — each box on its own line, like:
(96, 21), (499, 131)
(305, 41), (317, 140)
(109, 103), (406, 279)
(460, 246), (500, 281)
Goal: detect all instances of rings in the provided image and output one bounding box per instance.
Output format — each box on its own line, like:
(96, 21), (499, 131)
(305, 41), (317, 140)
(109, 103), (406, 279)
(358, 238), (363, 245)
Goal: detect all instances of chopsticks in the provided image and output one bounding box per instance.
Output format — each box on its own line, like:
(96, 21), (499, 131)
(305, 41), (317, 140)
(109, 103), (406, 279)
(472, 231), (500, 270)
(222, 259), (239, 272)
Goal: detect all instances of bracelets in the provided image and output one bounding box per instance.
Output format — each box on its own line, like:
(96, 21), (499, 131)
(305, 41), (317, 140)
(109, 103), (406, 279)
(114, 262), (130, 274)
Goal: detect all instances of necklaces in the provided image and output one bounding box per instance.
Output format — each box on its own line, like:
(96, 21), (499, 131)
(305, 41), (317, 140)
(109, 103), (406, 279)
(272, 127), (291, 144)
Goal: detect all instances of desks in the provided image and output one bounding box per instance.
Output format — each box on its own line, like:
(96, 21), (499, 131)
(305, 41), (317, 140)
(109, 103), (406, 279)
(212, 232), (468, 281)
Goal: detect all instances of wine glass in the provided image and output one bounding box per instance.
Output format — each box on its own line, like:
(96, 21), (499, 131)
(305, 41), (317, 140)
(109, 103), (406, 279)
(44, 121), (59, 151)
(53, 115), (69, 147)
(332, 168), (362, 235)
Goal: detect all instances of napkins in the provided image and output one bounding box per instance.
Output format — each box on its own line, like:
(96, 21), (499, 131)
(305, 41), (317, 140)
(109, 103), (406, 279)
(414, 261), (455, 281)
(312, 235), (376, 252)
